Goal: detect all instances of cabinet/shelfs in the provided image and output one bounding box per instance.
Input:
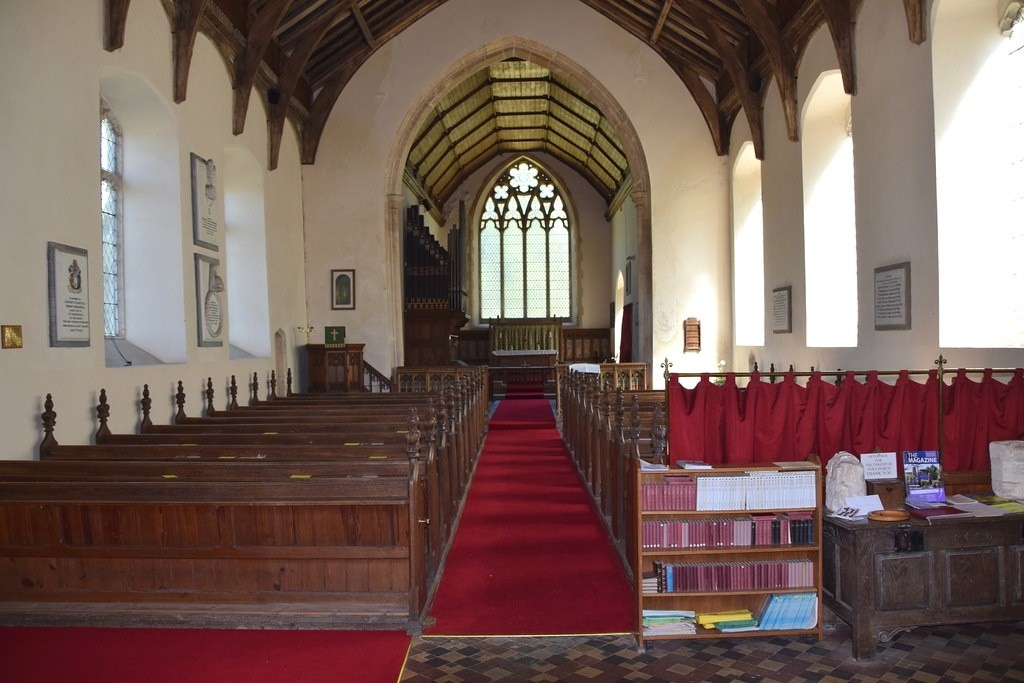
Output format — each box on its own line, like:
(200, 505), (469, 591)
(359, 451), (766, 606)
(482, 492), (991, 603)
(632, 453), (824, 650)
(822, 492), (1024, 662)
(307, 342), (365, 391)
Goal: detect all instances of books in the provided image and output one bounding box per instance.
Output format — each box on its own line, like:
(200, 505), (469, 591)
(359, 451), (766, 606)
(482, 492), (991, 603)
(676, 459), (714, 470)
(901, 449), (946, 503)
(638, 458), (670, 471)
(905, 493), (1024, 526)
(641, 470), (819, 637)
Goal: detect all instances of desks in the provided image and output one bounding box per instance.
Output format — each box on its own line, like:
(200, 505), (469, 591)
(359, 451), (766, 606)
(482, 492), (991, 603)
(491, 350), (558, 386)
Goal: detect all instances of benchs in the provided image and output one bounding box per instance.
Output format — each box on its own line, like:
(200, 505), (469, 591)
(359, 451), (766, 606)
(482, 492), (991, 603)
(175, 380), (465, 506)
(92, 386), (455, 536)
(138, 384), (461, 517)
(201, 366), (490, 499)
(38, 391), (451, 559)
(1, 406), (430, 640)
(559, 367), (667, 588)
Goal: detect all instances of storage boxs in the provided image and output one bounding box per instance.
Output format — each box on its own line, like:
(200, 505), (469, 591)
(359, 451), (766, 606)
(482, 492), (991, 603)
(866, 479), (905, 509)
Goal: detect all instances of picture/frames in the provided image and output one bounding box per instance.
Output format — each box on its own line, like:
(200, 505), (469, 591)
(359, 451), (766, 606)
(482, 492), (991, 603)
(772, 285), (792, 333)
(190, 152), (219, 252)
(47, 242), (91, 348)
(873, 262), (912, 331)
(331, 269), (356, 310)
(194, 253), (224, 347)
